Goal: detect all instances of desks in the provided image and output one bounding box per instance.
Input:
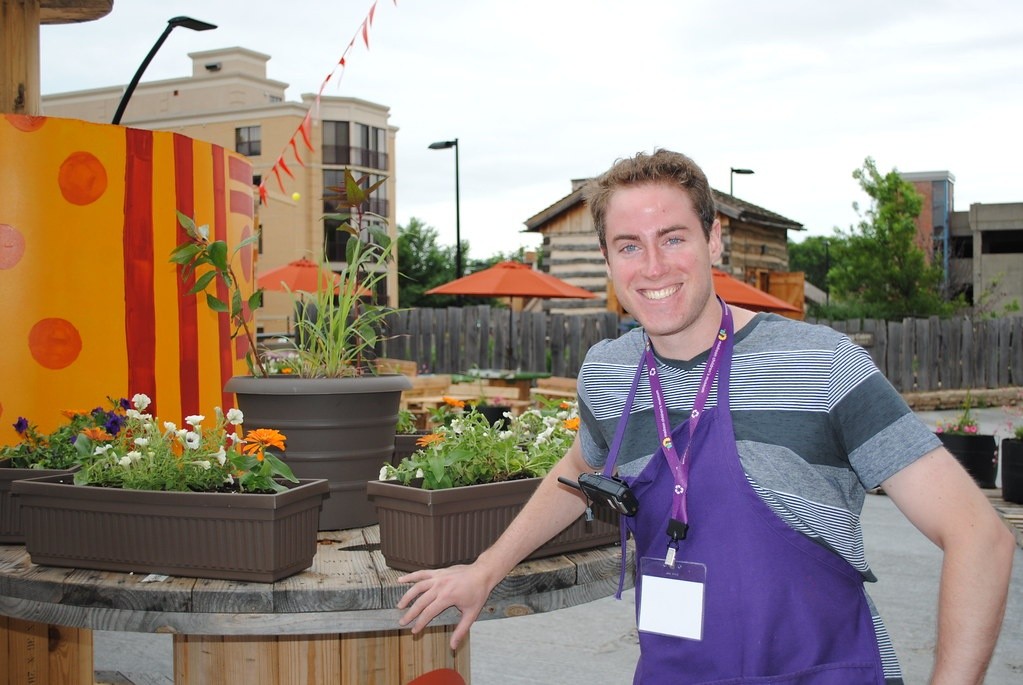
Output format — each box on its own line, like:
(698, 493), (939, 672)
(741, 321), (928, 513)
(0, 529), (630, 685)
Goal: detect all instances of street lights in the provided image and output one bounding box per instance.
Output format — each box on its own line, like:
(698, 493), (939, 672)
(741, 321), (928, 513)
(111, 16), (218, 125)
(730, 167), (754, 196)
(428, 139), (462, 279)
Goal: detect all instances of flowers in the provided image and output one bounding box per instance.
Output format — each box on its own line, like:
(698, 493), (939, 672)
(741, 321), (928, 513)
(991, 387), (1023, 466)
(376, 405), (575, 488)
(934, 384), (981, 434)
(0, 390), (301, 495)
(462, 370), (515, 406)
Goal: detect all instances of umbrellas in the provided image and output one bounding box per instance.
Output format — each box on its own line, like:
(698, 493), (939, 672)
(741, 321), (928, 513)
(255, 257), (372, 316)
(426, 261), (595, 369)
(710, 266), (803, 313)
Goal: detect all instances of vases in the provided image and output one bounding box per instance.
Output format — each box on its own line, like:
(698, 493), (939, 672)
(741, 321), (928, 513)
(16, 473), (328, 582)
(0, 440), (114, 543)
(1000, 436), (1023, 505)
(932, 433), (997, 489)
(464, 405), (513, 433)
(368, 482), (632, 571)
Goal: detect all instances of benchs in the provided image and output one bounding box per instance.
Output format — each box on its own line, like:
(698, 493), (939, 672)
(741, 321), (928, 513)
(373, 356), (578, 431)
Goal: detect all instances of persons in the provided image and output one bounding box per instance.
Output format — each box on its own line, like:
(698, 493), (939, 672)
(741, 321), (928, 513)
(397, 149), (1016, 685)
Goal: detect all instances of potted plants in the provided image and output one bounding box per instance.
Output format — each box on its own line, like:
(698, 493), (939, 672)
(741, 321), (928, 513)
(168, 158), (407, 532)
